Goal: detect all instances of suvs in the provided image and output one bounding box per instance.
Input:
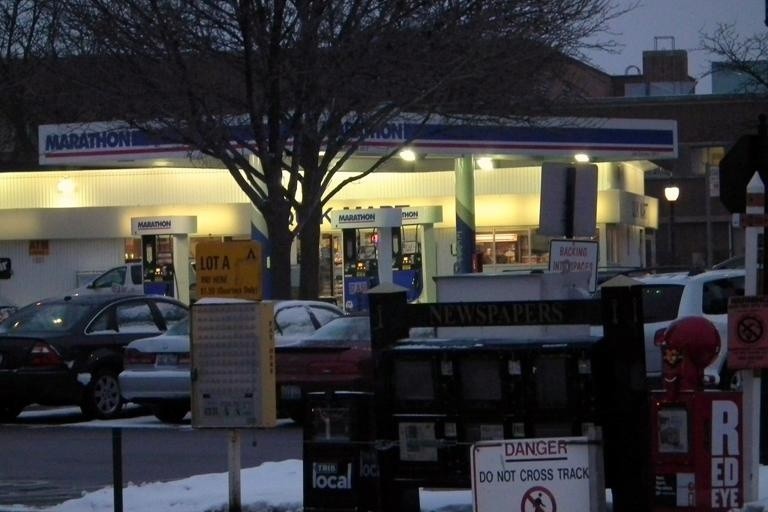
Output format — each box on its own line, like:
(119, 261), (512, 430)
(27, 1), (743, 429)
(582, 266), (746, 390)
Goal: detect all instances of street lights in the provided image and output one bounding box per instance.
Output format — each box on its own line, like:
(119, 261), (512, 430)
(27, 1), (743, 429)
(662, 174), (680, 266)
